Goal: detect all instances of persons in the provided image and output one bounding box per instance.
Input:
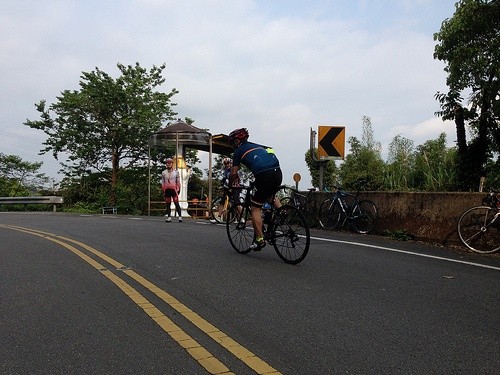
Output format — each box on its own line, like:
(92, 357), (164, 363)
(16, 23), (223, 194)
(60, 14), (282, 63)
(210, 158), (246, 224)
(162, 157), (182, 222)
(227, 127), (287, 250)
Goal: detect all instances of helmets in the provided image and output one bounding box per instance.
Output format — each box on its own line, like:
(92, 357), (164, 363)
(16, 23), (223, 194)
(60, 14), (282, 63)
(166, 158), (174, 163)
(224, 158), (233, 164)
(227, 128), (249, 142)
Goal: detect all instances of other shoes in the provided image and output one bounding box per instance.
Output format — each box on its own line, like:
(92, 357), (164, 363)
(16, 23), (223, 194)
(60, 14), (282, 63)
(210, 219), (218, 223)
(250, 238), (266, 249)
(240, 218), (245, 223)
(166, 216), (172, 222)
(178, 216), (183, 221)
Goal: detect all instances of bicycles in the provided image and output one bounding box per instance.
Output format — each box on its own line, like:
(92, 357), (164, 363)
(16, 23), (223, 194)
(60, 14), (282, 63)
(210, 181), (250, 225)
(456, 177), (500, 254)
(277, 183), (323, 228)
(226, 184), (311, 265)
(317, 184), (379, 235)
